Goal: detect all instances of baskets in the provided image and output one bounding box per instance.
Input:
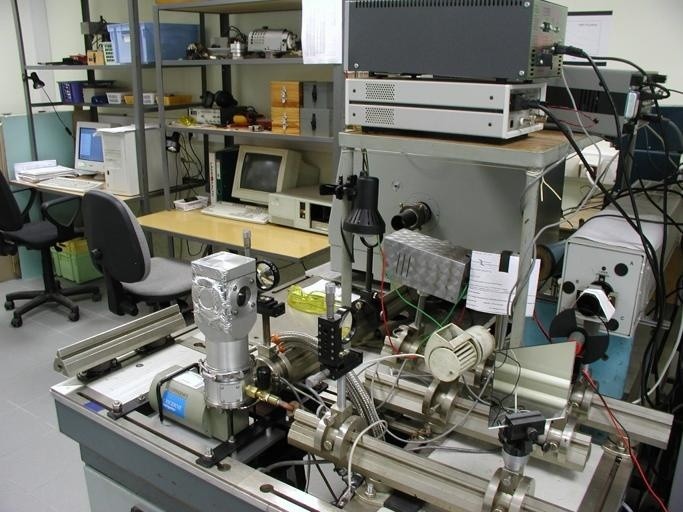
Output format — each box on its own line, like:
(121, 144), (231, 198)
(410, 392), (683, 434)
(56, 79), (194, 105)
(99, 41), (120, 66)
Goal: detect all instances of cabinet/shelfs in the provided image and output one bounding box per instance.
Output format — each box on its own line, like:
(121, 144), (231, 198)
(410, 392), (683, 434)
(152, 1), (343, 208)
(10, 2), (210, 194)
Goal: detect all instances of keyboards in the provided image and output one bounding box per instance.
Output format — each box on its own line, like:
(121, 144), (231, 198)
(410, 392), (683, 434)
(201, 199), (268, 226)
(36, 176), (104, 193)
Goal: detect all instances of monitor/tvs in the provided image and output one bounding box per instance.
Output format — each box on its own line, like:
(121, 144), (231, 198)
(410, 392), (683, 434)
(74, 120), (122, 180)
(230, 144), (321, 208)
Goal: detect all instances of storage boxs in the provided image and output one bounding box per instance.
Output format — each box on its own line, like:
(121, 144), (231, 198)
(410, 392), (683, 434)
(50, 248), (104, 284)
(107, 23), (203, 64)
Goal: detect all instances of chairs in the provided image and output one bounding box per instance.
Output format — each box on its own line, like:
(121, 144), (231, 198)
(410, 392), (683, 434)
(82, 188), (194, 315)
(0, 171), (101, 328)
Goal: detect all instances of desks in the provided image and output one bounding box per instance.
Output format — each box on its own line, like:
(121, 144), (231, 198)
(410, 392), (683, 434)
(134, 198), (331, 280)
(333, 127), (602, 339)
(7, 174), (219, 295)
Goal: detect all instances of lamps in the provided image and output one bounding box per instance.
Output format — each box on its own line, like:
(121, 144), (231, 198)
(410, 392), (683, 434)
(22, 71), (44, 90)
(318, 173), (384, 236)
(164, 130), (181, 152)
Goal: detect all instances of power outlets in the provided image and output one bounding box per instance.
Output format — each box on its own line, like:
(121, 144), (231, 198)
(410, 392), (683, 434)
(185, 131), (198, 138)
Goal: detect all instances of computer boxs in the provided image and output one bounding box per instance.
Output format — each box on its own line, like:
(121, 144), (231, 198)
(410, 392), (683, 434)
(269, 182), (331, 235)
(102, 121), (184, 197)
